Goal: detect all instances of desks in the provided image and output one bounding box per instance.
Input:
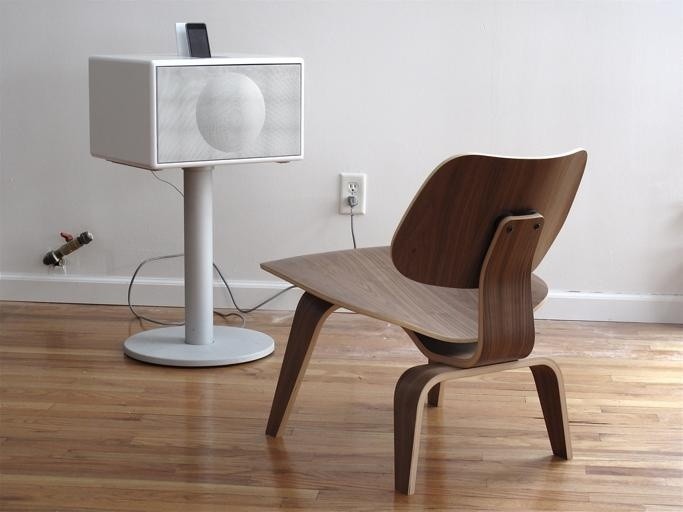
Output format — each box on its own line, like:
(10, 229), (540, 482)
(87, 54), (304, 370)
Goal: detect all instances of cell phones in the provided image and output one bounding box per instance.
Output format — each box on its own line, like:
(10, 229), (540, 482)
(184, 23), (211, 58)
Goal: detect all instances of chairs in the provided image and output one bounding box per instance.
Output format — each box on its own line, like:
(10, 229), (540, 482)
(257, 148), (590, 497)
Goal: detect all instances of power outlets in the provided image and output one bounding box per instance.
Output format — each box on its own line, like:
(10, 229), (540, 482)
(339, 172), (366, 216)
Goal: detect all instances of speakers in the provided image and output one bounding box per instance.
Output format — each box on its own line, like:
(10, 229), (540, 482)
(87, 51), (305, 172)
